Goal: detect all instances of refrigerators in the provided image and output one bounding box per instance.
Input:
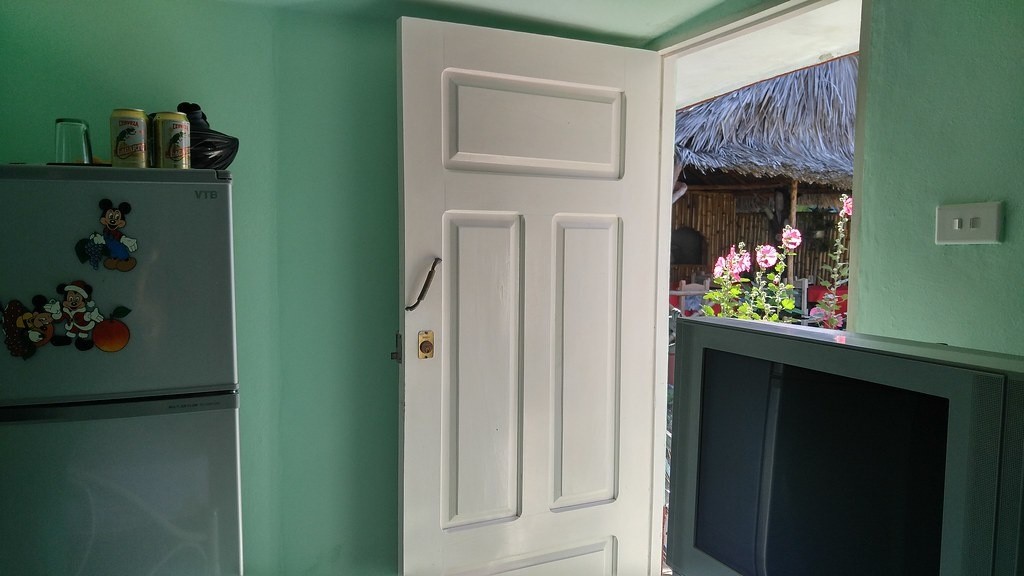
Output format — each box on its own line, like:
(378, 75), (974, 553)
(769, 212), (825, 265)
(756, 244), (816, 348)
(1, 162), (244, 575)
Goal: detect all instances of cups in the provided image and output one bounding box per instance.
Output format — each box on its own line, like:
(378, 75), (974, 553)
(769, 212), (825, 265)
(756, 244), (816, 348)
(52, 119), (91, 163)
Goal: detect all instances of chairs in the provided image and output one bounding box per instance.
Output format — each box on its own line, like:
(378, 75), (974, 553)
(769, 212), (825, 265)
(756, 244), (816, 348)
(677, 279), (710, 317)
(793, 275), (818, 326)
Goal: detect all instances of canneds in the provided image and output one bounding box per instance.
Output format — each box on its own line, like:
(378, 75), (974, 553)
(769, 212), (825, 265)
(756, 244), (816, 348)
(109, 108), (191, 170)
